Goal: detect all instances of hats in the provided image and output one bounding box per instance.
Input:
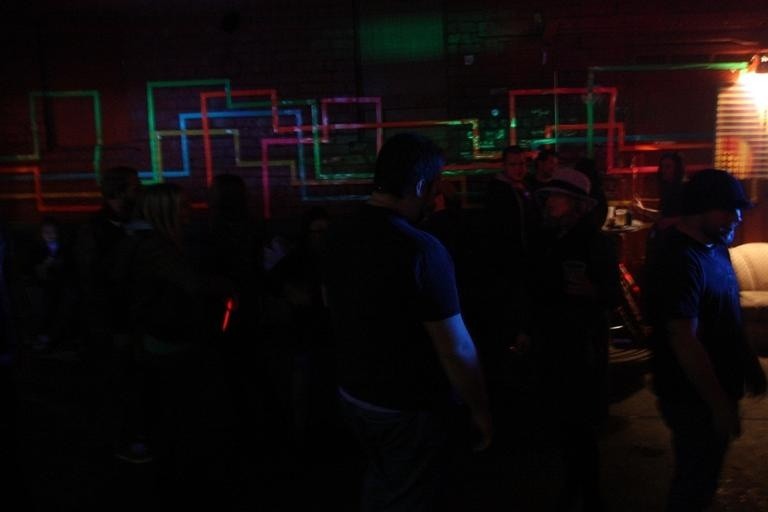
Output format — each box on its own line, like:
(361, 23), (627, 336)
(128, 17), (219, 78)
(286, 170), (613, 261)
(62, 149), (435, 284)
(534, 171), (591, 203)
(687, 168), (753, 214)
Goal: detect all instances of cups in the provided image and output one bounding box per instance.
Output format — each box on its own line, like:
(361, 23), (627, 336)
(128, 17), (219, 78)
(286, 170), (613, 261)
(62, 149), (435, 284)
(614, 209), (627, 227)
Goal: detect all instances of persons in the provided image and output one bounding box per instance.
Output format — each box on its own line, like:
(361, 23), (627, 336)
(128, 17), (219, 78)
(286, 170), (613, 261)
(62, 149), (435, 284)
(1, 131), (622, 511)
(640, 169), (767, 511)
(653, 152), (686, 213)
